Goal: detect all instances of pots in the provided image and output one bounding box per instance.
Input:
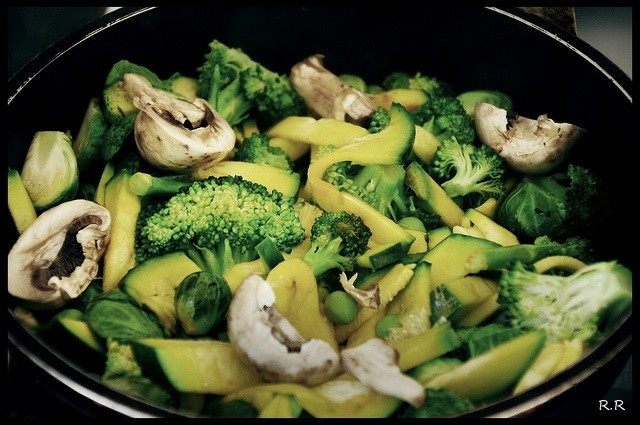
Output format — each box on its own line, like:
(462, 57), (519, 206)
(0, 2), (633, 424)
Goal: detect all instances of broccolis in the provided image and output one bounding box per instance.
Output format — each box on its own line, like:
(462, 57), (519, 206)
(7, 37), (633, 419)
